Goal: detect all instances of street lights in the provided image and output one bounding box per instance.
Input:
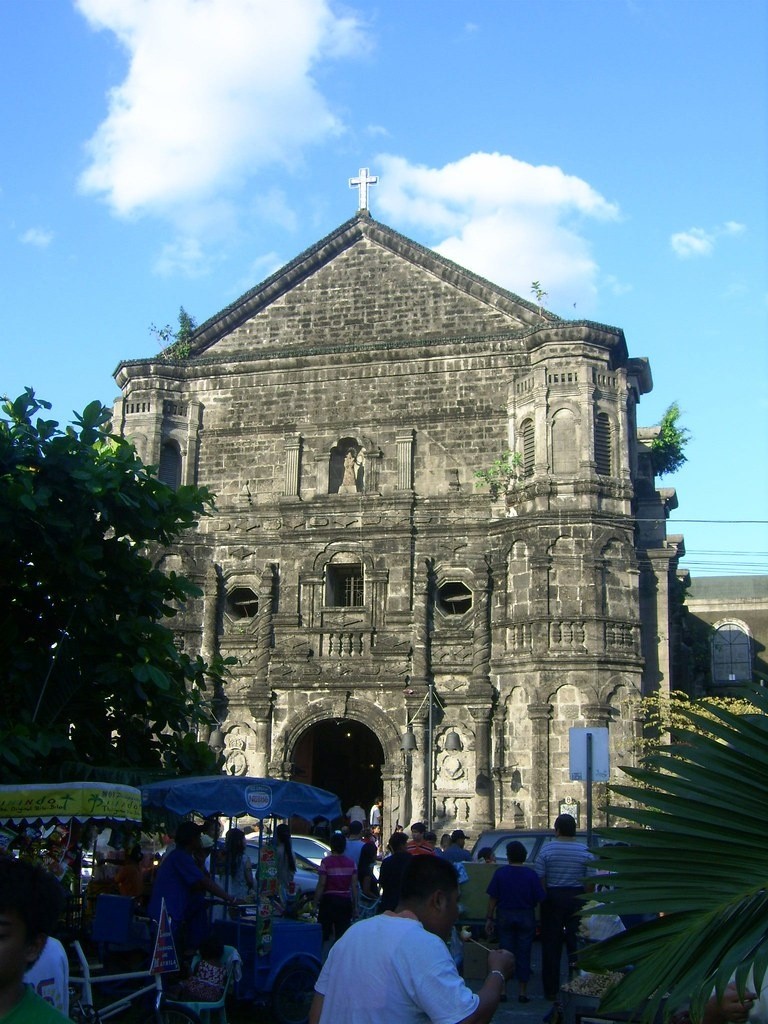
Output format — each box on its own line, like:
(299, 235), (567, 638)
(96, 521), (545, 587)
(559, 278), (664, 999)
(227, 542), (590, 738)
(400, 683), (463, 834)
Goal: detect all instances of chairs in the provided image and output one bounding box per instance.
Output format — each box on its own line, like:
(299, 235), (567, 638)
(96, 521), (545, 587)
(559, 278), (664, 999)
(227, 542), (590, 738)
(160, 945), (243, 1024)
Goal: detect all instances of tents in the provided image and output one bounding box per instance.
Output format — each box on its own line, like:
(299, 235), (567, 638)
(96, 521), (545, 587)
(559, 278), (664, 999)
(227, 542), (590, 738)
(0, 775), (344, 926)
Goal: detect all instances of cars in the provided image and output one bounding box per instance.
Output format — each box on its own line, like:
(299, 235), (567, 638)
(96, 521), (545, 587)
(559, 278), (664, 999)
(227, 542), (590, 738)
(246, 828), (383, 888)
(205, 837), (322, 924)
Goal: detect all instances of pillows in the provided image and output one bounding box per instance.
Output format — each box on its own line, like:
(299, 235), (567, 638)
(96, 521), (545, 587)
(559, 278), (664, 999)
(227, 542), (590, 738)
(184, 961), (228, 1001)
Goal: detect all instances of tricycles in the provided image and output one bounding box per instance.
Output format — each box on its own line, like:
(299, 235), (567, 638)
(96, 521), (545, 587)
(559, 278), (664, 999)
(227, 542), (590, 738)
(0, 771), (343, 1023)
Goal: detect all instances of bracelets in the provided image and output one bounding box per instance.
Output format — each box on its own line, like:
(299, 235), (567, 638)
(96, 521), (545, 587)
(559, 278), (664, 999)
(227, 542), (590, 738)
(231, 897), (236, 904)
(486, 915), (492, 920)
(488, 970), (506, 984)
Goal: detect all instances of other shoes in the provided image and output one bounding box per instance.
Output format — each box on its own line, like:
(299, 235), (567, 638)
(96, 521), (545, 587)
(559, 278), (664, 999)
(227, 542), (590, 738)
(500, 994), (507, 1002)
(518, 994), (530, 1003)
(544, 994), (556, 1001)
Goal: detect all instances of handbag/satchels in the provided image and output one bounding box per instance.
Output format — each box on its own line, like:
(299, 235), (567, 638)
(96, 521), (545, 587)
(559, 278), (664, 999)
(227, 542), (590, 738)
(186, 960), (227, 1000)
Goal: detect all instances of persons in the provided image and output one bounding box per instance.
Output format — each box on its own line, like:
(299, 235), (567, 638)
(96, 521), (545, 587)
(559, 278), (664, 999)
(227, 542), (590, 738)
(342, 451), (356, 487)
(0, 797), (768, 1024)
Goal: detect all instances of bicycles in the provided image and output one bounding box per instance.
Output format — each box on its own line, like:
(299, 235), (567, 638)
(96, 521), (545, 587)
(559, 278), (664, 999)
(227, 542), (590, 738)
(346, 880), (382, 929)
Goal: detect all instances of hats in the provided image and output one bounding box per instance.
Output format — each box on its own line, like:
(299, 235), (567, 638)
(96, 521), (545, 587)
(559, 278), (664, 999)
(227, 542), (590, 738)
(200, 833), (215, 848)
(452, 830), (470, 840)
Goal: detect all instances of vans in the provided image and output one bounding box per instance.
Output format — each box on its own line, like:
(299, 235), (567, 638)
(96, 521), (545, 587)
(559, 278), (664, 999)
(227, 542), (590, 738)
(470, 826), (631, 877)
